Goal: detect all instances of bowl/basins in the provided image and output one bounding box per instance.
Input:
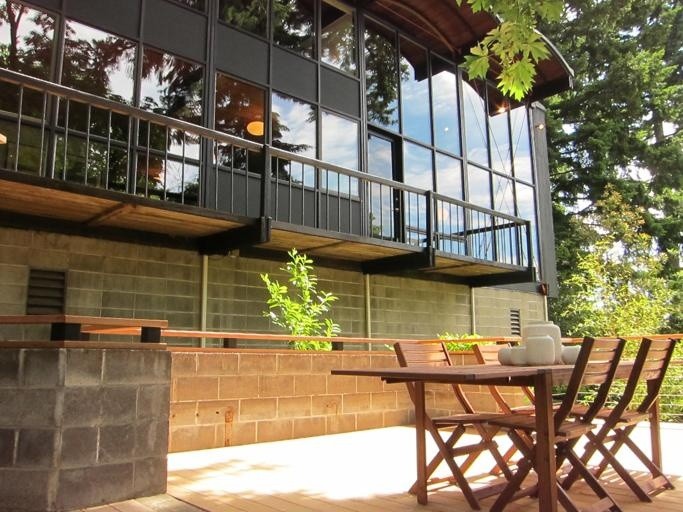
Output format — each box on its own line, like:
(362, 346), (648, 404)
(497, 346), (512, 365)
(510, 347), (525, 366)
(561, 345), (581, 365)
